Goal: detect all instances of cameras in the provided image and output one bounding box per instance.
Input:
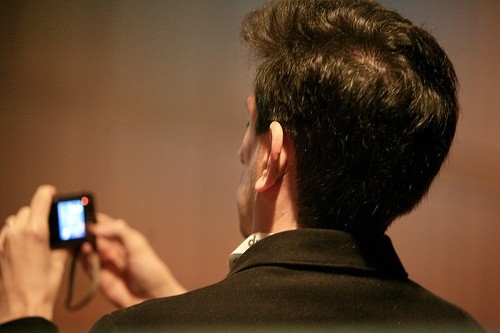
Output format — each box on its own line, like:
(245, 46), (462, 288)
(48, 191), (97, 248)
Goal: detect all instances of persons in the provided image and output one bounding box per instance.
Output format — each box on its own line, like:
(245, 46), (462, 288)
(0, 0), (488, 333)
